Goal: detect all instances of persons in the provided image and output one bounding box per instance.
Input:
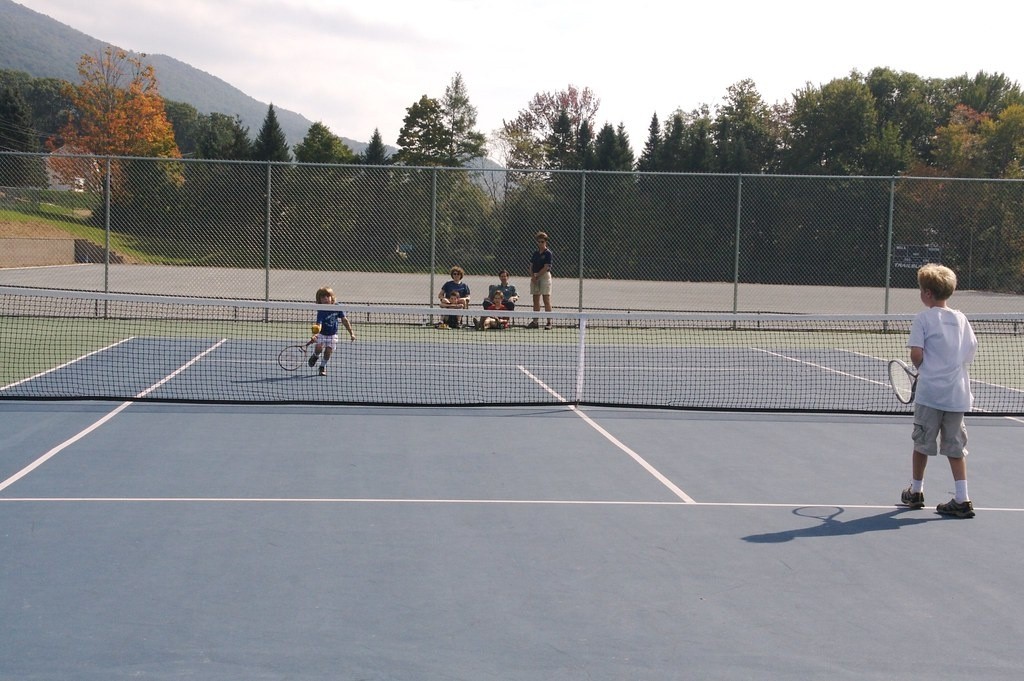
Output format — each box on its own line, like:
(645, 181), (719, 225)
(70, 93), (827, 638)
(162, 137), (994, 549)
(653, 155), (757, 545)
(900, 262), (979, 519)
(526, 231), (552, 330)
(308, 287), (356, 376)
(473, 270), (519, 331)
(435, 265), (470, 329)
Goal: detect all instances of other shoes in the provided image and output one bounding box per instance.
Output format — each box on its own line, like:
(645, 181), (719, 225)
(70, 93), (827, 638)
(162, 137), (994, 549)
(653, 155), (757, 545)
(435, 321), (443, 329)
(479, 318), (485, 331)
(456, 321), (463, 329)
(309, 354), (318, 367)
(319, 365), (327, 376)
(473, 317), (480, 331)
(545, 322), (553, 330)
(523, 321), (539, 329)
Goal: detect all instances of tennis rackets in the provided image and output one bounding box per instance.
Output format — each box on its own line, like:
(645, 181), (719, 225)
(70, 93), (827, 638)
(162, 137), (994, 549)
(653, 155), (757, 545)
(277, 340), (314, 372)
(887, 358), (919, 404)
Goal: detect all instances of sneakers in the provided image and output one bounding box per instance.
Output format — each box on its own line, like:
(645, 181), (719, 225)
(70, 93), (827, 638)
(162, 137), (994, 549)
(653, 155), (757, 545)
(936, 492), (975, 518)
(901, 483), (926, 508)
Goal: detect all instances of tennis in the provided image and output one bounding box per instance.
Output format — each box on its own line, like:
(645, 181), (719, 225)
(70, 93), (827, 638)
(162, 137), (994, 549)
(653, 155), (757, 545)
(311, 324), (320, 334)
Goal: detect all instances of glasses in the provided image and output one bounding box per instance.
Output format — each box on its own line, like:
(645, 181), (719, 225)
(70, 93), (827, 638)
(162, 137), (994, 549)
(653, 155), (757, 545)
(537, 241), (545, 243)
(453, 273), (460, 275)
